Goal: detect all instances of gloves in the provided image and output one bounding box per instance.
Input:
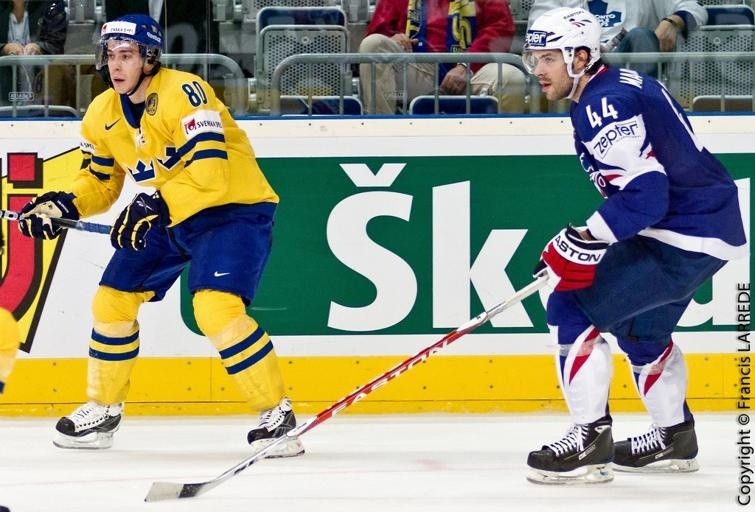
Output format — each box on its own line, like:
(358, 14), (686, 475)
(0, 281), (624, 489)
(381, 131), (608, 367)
(110, 190), (172, 253)
(18, 190), (80, 241)
(531, 221), (610, 292)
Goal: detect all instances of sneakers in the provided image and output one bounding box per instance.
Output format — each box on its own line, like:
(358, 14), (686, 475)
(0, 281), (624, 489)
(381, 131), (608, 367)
(615, 416), (698, 468)
(55, 401), (124, 437)
(527, 413), (615, 473)
(247, 394), (296, 447)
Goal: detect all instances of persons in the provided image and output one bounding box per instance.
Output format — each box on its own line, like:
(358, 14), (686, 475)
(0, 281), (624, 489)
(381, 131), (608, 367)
(12, 12), (298, 443)
(256, 1), (355, 98)
(358, 2), (528, 115)
(524, 0), (709, 117)
(521, 6), (749, 475)
(0, 0), (69, 108)
(90, 1), (228, 114)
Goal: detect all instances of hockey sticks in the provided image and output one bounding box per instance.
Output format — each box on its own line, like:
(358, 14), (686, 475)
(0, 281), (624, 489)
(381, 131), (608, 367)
(144, 273), (546, 501)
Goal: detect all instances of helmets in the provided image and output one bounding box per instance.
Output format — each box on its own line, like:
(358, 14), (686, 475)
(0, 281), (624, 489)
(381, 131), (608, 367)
(96, 13), (165, 77)
(522, 6), (602, 78)
